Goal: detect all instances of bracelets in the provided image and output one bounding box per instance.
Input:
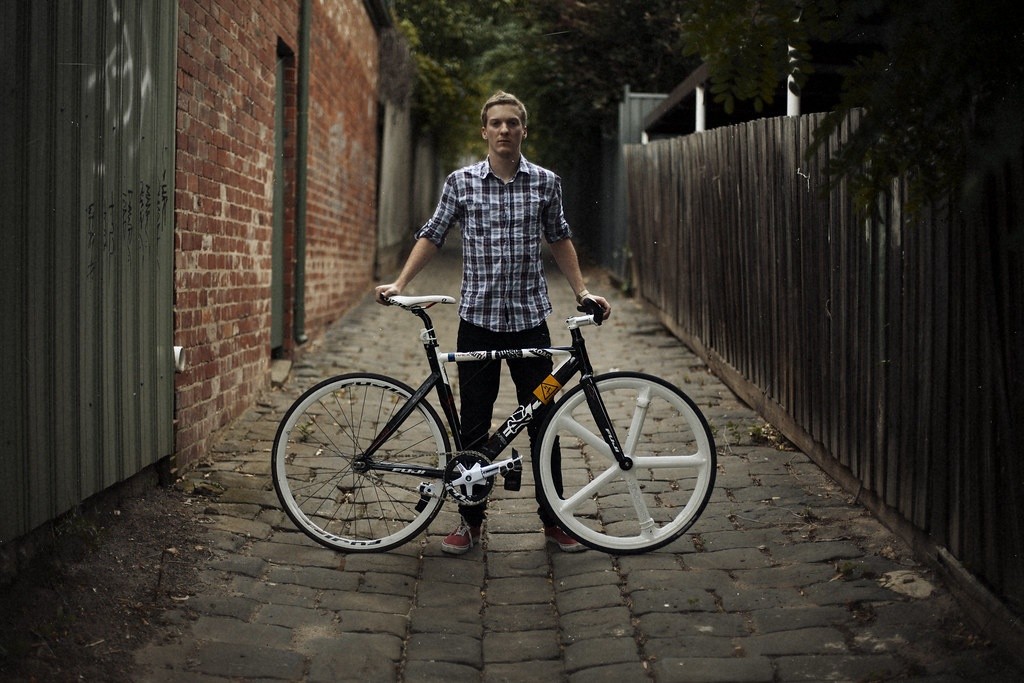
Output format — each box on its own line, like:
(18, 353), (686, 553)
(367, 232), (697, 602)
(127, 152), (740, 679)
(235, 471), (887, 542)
(576, 290), (589, 303)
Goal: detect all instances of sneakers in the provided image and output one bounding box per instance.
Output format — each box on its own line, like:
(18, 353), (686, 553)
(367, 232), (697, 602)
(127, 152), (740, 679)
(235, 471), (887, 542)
(441, 515), (481, 555)
(543, 525), (589, 553)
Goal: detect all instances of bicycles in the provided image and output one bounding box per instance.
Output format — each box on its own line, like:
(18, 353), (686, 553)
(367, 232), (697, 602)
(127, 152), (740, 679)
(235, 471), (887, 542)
(271, 294), (718, 555)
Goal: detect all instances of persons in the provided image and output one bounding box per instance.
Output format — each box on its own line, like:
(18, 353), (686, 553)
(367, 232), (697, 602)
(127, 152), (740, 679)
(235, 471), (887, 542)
(376, 90), (611, 554)
